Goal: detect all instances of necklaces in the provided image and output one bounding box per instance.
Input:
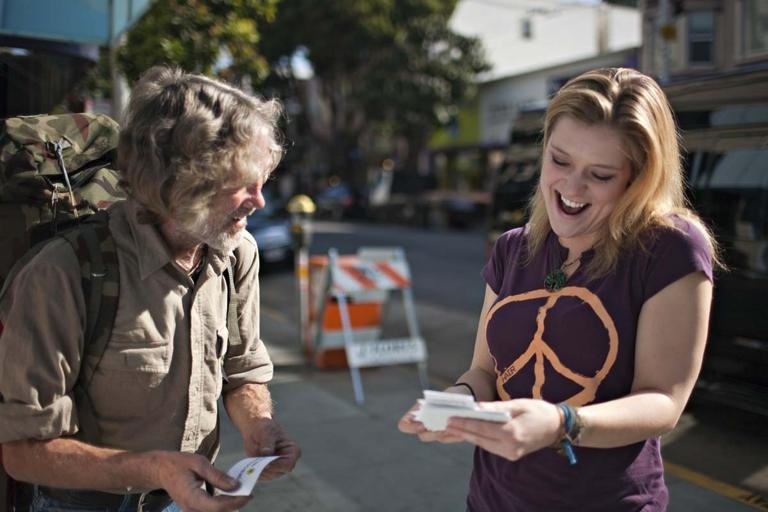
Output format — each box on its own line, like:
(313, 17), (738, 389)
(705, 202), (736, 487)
(542, 237), (603, 293)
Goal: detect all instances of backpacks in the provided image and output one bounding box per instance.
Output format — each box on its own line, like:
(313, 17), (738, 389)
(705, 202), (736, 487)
(0, 111), (139, 433)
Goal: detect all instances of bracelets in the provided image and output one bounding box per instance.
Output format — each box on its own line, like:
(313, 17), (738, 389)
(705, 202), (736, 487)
(556, 402), (584, 469)
(451, 379), (478, 403)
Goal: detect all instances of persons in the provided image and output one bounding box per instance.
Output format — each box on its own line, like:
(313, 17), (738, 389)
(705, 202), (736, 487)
(0, 66), (302, 510)
(396, 65), (730, 512)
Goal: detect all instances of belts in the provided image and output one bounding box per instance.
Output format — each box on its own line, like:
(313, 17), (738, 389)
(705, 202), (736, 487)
(38, 487), (174, 512)
